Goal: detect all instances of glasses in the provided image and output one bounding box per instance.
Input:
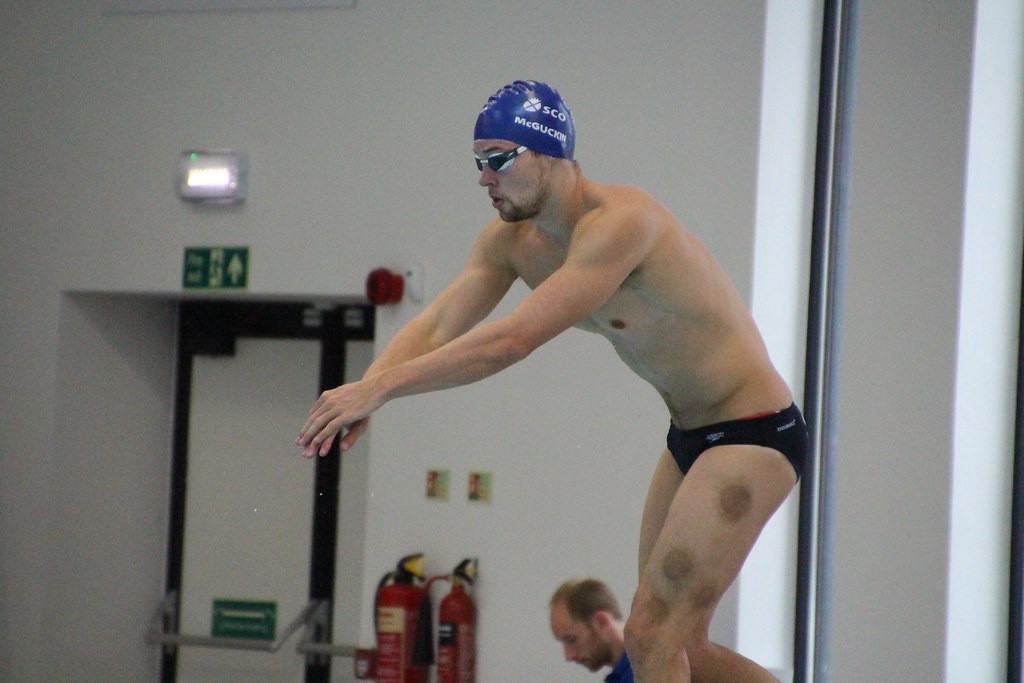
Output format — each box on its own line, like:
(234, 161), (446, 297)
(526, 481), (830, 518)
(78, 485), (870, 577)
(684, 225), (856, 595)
(475, 146), (528, 172)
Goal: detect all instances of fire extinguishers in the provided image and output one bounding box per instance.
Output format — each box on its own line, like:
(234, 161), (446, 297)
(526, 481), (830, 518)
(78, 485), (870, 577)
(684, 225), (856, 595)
(371, 551), (428, 683)
(412, 558), (479, 683)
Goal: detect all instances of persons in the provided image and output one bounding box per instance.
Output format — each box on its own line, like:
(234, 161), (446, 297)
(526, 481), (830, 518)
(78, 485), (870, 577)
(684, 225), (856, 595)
(293, 79), (809, 683)
(549, 577), (634, 683)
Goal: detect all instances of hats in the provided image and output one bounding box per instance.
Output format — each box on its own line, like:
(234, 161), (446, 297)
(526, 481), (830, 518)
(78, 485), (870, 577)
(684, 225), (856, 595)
(475, 79), (575, 161)
